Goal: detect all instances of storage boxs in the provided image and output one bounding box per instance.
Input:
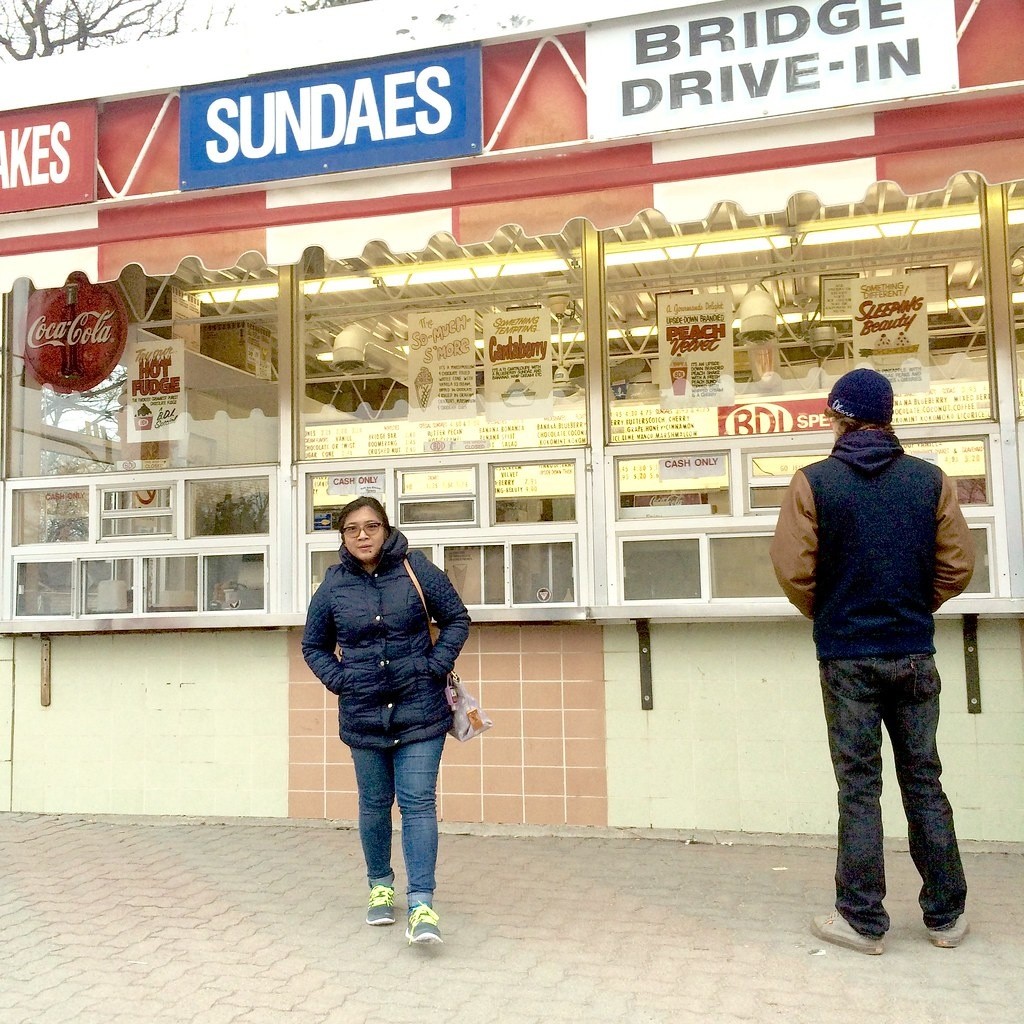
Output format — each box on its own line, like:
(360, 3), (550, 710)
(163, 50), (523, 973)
(201, 321), (273, 382)
(147, 286), (205, 354)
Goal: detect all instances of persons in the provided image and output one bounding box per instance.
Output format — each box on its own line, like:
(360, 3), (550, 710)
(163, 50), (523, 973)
(301, 496), (471, 944)
(769, 367), (979, 956)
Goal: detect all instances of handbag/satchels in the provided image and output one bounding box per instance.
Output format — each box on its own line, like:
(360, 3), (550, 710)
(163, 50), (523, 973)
(445, 670), (492, 742)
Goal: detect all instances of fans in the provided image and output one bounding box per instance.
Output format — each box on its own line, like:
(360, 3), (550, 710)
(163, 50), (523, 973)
(548, 295), (646, 397)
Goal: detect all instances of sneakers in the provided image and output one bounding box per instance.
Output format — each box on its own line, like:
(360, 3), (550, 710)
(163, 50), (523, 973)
(366, 884), (395, 925)
(928, 915), (968, 947)
(405, 900), (443, 946)
(810, 910), (884, 955)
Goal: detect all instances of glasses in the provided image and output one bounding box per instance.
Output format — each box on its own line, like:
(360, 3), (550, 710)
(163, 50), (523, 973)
(342, 522), (383, 538)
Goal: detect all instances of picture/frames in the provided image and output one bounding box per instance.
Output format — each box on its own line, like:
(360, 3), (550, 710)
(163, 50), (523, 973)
(620, 494), (707, 507)
(496, 499), (555, 523)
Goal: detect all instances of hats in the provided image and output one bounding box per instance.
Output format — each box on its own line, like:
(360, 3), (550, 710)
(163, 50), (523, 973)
(827, 368), (893, 423)
